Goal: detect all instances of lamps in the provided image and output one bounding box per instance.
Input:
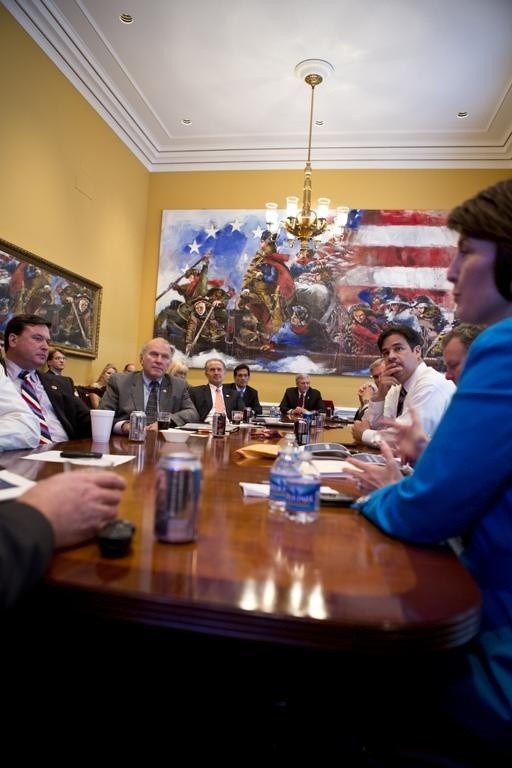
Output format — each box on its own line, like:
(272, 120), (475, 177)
(264, 58), (351, 260)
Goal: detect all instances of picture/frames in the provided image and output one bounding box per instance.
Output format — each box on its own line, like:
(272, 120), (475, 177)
(0, 237), (103, 359)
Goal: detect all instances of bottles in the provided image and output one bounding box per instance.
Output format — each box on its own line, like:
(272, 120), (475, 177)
(269, 434), (322, 524)
(270, 405), (281, 419)
(315, 414), (323, 433)
(260, 510), (319, 585)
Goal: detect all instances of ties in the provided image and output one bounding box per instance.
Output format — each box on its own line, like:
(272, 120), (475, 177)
(215, 388), (224, 413)
(298, 393), (304, 407)
(397, 385), (407, 417)
(17, 370), (52, 447)
(145, 381), (158, 426)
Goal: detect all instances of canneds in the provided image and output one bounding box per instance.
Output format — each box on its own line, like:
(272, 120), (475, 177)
(153, 451), (202, 544)
(127, 443), (145, 475)
(243, 407), (253, 424)
(294, 418), (309, 446)
(127, 411), (147, 441)
(315, 416), (324, 431)
(212, 412), (226, 438)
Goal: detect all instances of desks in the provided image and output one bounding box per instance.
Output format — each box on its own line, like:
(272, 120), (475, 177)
(0, 419), (483, 656)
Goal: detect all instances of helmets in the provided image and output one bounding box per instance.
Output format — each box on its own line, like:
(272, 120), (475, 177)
(186, 267), (201, 279)
(386, 294), (410, 307)
(261, 229), (281, 246)
(375, 285), (392, 303)
(412, 295), (435, 307)
(292, 305), (308, 323)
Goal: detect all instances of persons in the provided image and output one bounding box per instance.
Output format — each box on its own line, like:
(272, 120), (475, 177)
(350, 322), (459, 474)
(441, 320), (489, 388)
(353, 356), (391, 436)
(1, 313), (147, 449)
(44, 336), (327, 462)
(342, 179), (512, 729)
(0, 359), (42, 452)
(1, 464), (129, 611)
(0, 250), (97, 356)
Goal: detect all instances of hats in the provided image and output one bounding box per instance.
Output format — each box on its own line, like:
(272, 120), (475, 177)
(190, 295), (209, 307)
(208, 286), (225, 295)
(348, 303), (372, 319)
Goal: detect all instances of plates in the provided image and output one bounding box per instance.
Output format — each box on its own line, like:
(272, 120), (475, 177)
(159, 430), (197, 442)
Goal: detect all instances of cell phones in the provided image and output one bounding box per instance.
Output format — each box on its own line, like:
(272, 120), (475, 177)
(320, 492), (354, 506)
(60, 448), (102, 459)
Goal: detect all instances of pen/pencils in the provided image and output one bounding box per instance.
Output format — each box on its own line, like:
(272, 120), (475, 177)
(60, 451), (103, 458)
(174, 427), (198, 432)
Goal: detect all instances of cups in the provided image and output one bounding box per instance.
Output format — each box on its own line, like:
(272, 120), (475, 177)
(157, 412), (171, 432)
(303, 413), (312, 429)
(91, 410), (115, 443)
(62, 459), (115, 476)
(232, 411), (242, 424)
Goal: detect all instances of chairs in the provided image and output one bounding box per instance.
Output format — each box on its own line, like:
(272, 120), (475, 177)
(320, 399), (334, 417)
